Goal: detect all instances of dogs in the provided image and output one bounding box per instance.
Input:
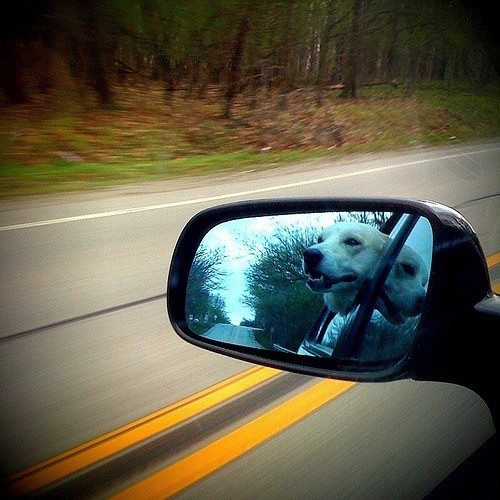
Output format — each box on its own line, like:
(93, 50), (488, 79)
(376, 245), (430, 326)
(302, 221), (392, 317)
